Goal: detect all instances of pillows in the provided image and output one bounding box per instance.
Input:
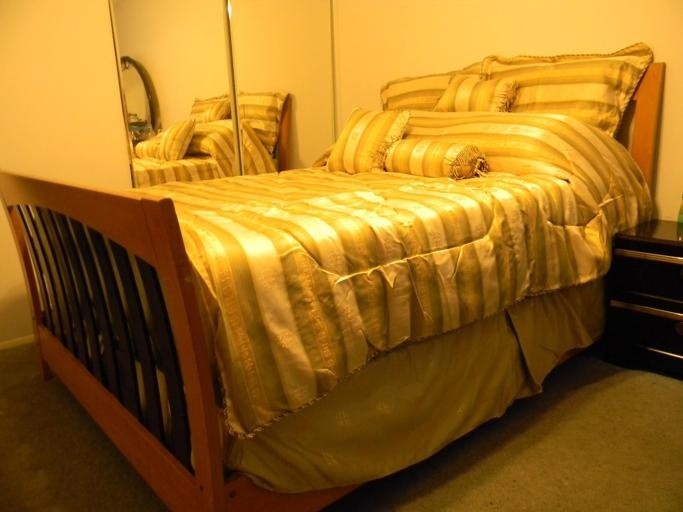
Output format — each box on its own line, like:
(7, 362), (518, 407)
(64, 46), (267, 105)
(133, 93), (289, 158)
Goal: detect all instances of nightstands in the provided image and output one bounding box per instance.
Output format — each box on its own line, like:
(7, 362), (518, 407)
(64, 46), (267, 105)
(602, 219), (682, 383)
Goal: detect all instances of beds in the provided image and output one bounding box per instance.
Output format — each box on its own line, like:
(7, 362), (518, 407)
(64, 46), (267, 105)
(0, 56), (666, 511)
(131, 92), (290, 188)
